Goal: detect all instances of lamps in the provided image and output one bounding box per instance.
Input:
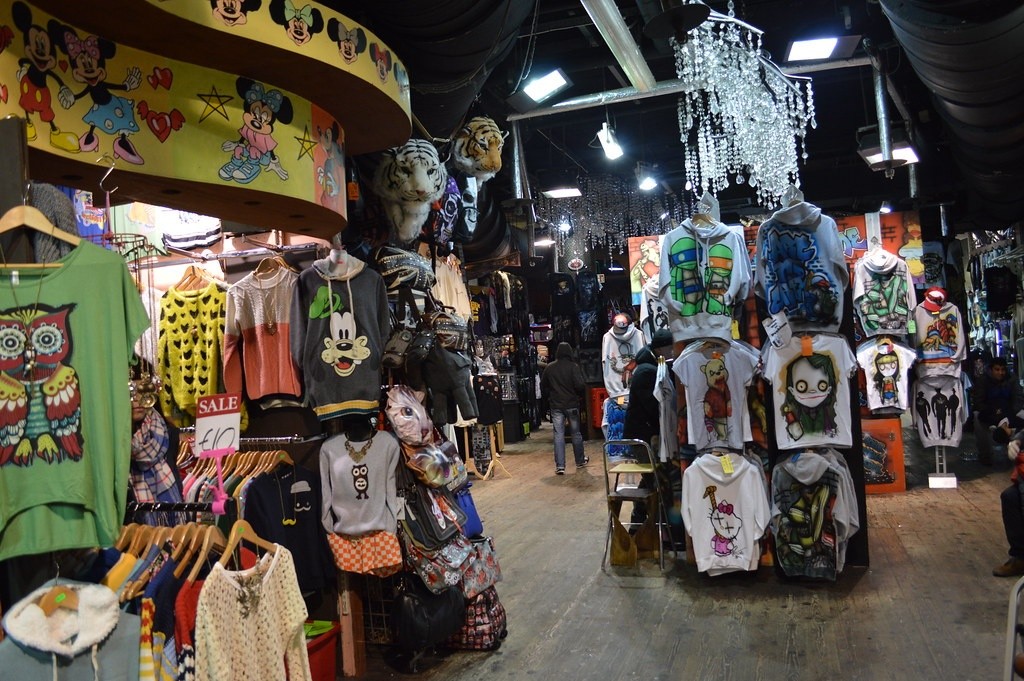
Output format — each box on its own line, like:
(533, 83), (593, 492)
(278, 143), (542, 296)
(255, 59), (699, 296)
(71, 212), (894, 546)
(880, 200), (891, 212)
(507, 0), (922, 197)
(608, 260), (624, 270)
(534, 229), (555, 246)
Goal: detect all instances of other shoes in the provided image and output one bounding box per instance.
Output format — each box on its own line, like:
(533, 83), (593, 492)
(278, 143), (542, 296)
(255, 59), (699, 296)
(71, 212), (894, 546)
(992, 556), (1024, 577)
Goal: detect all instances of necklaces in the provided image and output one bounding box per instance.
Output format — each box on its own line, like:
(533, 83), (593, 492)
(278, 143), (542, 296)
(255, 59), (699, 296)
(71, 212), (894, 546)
(258, 268), (279, 335)
(230, 533), (262, 619)
(274, 464), (297, 525)
(345, 426), (373, 464)
(183, 280), (202, 336)
(0, 225), (55, 398)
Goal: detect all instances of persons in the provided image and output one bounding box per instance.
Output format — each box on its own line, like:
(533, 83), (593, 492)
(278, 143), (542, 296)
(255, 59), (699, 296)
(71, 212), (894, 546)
(122, 352), (191, 526)
(623, 328), (674, 536)
(973, 356), (1024, 576)
(542, 342), (588, 474)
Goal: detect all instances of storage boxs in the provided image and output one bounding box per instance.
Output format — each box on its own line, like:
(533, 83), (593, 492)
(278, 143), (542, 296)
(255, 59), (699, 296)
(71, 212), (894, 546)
(304, 621), (339, 681)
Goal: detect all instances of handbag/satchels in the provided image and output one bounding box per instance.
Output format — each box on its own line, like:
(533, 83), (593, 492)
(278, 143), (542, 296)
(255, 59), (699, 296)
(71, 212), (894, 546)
(381, 283), (509, 651)
(375, 239), (437, 292)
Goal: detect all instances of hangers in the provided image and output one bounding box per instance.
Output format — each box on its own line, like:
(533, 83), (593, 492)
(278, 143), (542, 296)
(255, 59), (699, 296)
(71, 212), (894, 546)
(605, 198), (1024, 457)
(0, 180), (469, 622)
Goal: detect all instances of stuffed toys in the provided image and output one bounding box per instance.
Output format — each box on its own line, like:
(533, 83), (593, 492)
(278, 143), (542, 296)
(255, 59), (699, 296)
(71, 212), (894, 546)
(373, 139), (447, 244)
(450, 116), (507, 183)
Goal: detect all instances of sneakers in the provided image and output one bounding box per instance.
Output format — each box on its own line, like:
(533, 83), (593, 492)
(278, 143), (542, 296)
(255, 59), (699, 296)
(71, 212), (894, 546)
(576, 455), (589, 468)
(555, 466), (565, 474)
